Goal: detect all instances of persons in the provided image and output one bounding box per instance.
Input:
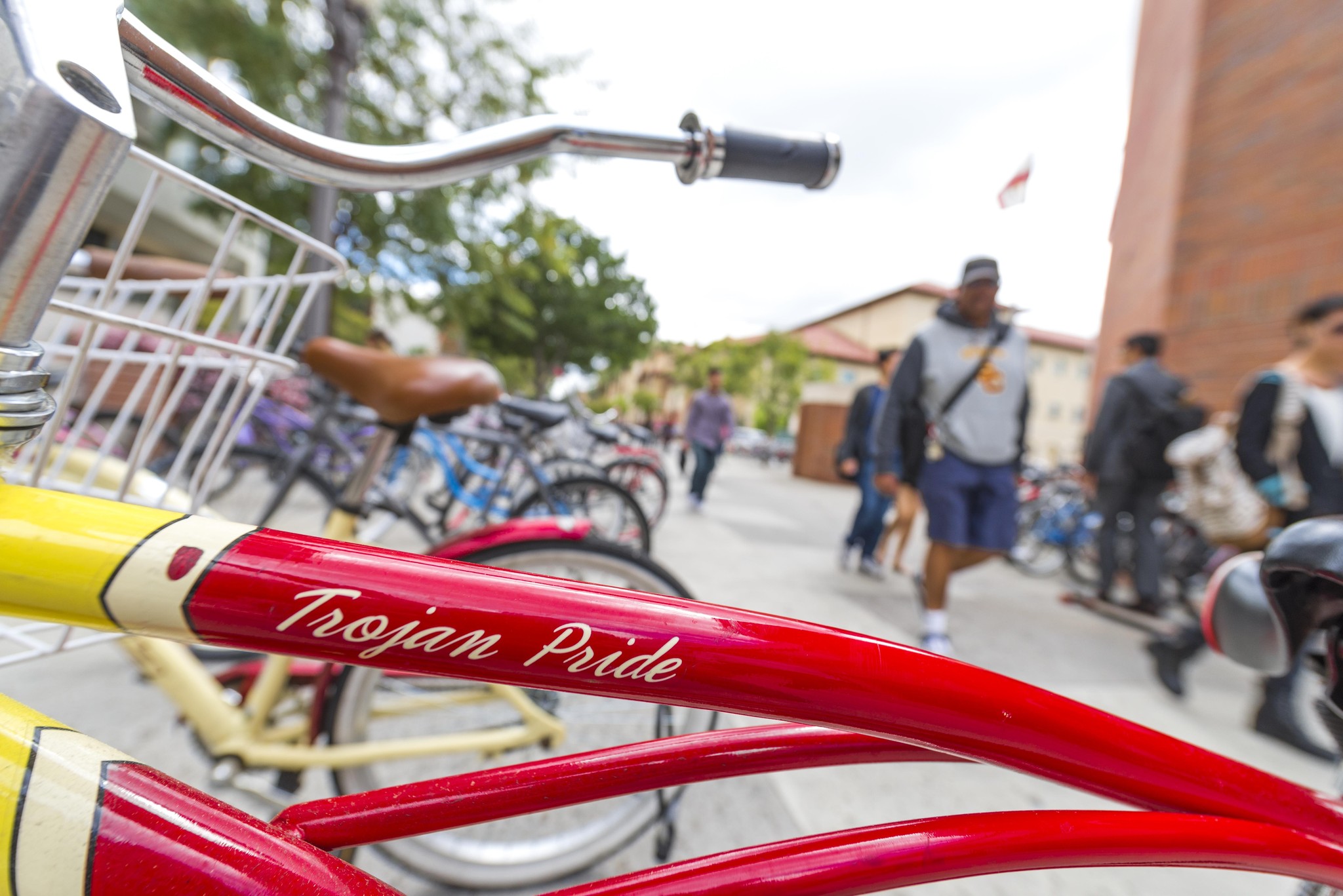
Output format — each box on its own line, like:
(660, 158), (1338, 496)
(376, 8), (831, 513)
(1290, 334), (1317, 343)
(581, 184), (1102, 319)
(870, 400), (925, 578)
(835, 347), (907, 582)
(681, 365), (735, 513)
(870, 255), (1032, 662)
(675, 389), (697, 479)
(1141, 292), (1342, 761)
(1148, 411), (1245, 621)
(1075, 328), (1187, 620)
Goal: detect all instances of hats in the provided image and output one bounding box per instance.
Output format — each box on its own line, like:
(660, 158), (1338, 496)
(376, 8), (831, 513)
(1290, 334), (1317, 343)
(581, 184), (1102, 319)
(961, 258), (999, 286)
(1298, 294), (1343, 324)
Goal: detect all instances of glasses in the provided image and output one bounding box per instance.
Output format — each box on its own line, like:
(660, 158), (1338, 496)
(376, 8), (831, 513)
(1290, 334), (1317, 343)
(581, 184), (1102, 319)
(1327, 325), (1343, 334)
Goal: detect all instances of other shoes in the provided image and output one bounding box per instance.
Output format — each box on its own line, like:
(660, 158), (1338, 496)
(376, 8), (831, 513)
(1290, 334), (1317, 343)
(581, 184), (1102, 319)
(912, 574), (927, 610)
(920, 634), (956, 659)
(859, 565), (883, 582)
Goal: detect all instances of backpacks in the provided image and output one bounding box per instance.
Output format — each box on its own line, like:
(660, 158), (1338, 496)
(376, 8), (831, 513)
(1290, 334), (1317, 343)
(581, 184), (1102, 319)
(1161, 361), (1306, 551)
(1114, 377), (1205, 482)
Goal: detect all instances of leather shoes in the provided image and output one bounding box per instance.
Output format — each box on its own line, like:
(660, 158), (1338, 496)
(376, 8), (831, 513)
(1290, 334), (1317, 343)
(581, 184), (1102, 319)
(1255, 702), (1336, 761)
(1148, 641), (1182, 694)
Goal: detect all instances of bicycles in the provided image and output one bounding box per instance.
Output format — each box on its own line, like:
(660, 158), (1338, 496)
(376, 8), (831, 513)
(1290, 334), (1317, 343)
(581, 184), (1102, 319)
(0, 0), (1343, 896)
